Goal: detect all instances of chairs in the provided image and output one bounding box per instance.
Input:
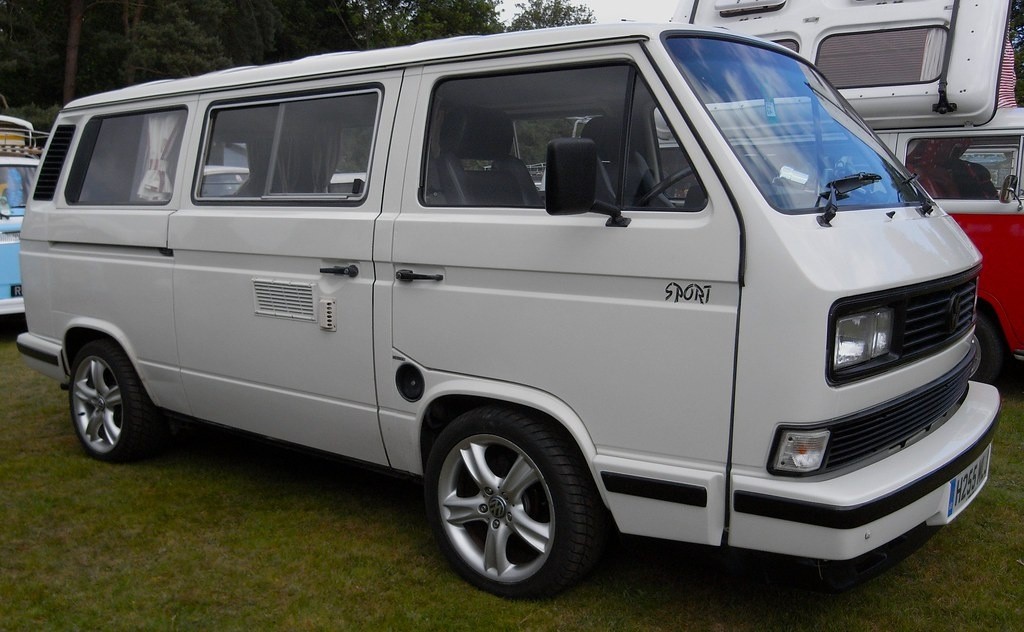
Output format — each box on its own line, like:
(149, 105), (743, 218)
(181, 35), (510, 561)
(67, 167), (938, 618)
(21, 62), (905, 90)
(580, 115), (678, 210)
(439, 107), (541, 207)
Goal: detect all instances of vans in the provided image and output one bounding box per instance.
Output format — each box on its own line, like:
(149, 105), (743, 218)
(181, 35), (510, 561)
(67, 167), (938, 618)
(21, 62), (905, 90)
(14, 19), (1000, 601)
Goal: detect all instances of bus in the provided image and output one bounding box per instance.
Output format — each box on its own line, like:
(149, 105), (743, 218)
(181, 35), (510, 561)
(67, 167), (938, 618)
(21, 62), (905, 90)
(653, 0), (1024, 404)
(0, 115), (51, 316)
(325, 171), (367, 192)
(199, 164), (250, 196)
